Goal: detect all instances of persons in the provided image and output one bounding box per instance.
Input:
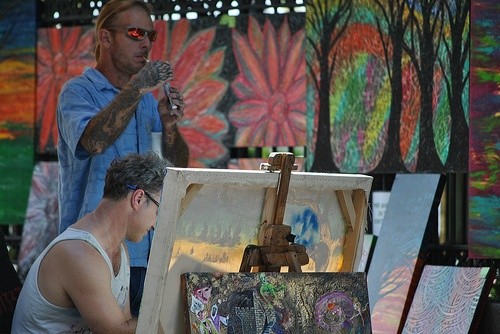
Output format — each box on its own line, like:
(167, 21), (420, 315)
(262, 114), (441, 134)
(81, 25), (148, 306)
(10, 150), (166, 334)
(51, 1), (186, 320)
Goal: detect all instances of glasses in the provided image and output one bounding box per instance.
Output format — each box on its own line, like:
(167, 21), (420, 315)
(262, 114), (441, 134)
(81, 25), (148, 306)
(104, 25), (158, 42)
(127, 184), (160, 207)
